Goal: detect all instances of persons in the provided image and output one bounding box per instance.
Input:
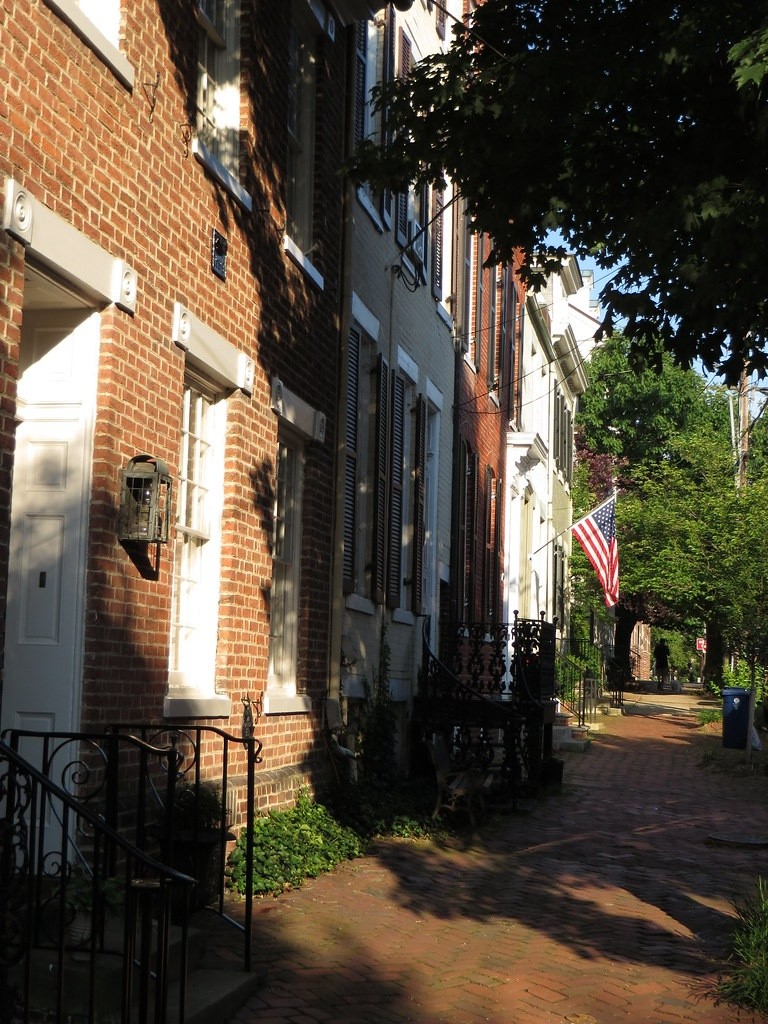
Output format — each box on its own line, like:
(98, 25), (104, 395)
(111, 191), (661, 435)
(654, 639), (670, 689)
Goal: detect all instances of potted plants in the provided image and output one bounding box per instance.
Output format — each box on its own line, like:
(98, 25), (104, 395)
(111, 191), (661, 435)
(155, 787), (236, 906)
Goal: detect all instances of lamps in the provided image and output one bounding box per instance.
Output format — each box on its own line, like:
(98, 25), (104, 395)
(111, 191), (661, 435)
(118, 454), (171, 543)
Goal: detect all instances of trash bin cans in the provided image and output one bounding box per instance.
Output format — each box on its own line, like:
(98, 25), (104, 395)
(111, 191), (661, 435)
(720, 687), (751, 751)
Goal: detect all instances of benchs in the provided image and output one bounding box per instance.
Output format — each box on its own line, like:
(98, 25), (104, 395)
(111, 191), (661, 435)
(421, 731), (494, 826)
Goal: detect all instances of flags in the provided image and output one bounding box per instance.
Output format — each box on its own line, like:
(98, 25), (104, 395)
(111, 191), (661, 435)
(569, 496), (619, 608)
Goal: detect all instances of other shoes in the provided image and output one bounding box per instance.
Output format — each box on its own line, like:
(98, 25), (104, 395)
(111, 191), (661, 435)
(658, 685), (660, 689)
(661, 687), (664, 691)
